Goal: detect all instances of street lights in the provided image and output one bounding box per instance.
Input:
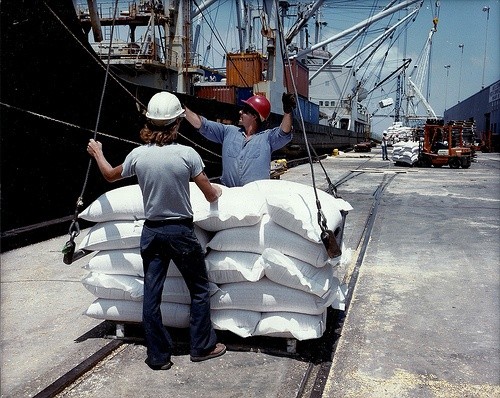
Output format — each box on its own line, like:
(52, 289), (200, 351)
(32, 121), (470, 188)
(444, 64), (451, 111)
(458, 43), (464, 103)
(481, 6), (490, 89)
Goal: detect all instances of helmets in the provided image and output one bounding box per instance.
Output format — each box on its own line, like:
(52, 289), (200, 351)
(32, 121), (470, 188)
(145, 91), (186, 121)
(241, 95), (271, 122)
(383, 130), (387, 133)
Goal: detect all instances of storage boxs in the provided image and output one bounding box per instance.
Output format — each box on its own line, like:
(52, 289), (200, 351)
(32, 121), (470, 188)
(192, 53), (319, 123)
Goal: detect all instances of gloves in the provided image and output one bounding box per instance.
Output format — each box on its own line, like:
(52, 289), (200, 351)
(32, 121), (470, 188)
(282, 92), (297, 114)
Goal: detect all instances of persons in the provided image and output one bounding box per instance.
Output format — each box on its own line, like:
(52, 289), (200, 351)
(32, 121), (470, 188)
(381, 131), (392, 160)
(393, 134), (400, 144)
(209, 72), (216, 82)
(182, 91), (296, 188)
(86, 91), (226, 370)
(430, 131), (449, 154)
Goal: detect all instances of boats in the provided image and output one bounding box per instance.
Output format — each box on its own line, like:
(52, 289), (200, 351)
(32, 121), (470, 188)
(1, 1), (440, 235)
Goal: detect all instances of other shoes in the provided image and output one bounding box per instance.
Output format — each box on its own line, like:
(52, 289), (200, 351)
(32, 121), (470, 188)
(190, 343), (227, 362)
(145, 357), (170, 370)
